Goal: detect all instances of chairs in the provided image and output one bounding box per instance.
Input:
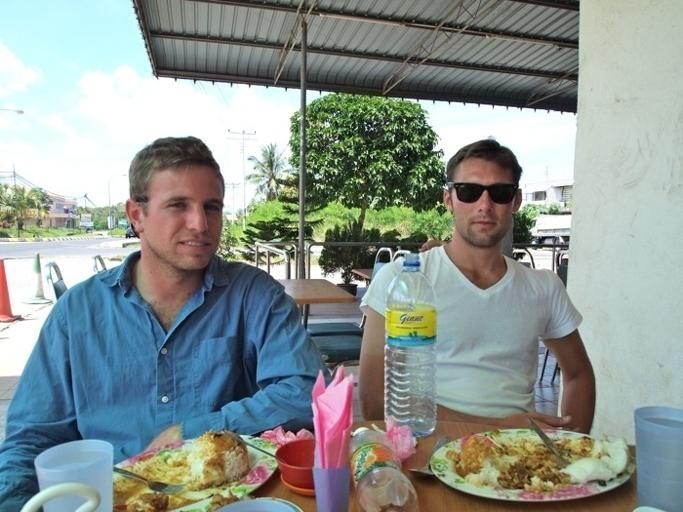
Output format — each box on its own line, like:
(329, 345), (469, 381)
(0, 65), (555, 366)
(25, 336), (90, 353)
(310, 250), (411, 367)
(304, 246), (393, 335)
(541, 252), (568, 385)
(44, 261), (68, 300)
(92, 255), (109, 274)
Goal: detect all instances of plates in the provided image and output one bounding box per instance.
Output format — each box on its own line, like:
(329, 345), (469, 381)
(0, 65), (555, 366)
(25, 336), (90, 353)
(431, 428), (636, 503)
(213, 498), (304, 511)
(280, 473), (314, 497)
(116, 431), (280, 512)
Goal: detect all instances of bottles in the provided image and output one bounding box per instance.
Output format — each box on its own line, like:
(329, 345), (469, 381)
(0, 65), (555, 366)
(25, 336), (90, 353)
(350, 422), (418, 511)
(382, 253), (438, 439)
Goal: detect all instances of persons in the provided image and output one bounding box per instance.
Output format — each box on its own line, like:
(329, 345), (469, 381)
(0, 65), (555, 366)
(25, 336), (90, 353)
(0, 136), (329, 512)
(358, 140), (595, 434)
(419, 237), (451, 252)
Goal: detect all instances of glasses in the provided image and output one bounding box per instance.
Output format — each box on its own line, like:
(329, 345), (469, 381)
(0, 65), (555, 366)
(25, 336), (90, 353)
(447, 183), (519, 204)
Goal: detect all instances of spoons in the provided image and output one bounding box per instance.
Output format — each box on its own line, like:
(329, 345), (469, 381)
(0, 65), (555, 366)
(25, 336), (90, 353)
(407, 435), (452, 482)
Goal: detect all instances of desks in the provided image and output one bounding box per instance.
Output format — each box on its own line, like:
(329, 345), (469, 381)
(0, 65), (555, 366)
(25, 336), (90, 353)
(351, 269), (373, 328)
(276, 278), (357, 329)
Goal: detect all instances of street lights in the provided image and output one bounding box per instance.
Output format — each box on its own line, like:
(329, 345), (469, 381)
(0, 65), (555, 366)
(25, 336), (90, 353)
(107, 173), (127, 231)
(0, 107), (25, 114)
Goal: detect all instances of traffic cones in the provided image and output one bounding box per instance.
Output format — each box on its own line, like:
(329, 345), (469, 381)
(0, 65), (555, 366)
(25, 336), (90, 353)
(0, 257), (23, 324)
(19, 252), (52, 305)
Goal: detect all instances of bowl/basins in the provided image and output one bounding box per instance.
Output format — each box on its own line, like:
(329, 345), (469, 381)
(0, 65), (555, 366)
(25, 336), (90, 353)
(274, 439), (317, 488)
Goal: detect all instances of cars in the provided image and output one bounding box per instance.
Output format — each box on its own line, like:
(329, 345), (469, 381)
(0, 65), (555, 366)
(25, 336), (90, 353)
(126, 226), (137, 238)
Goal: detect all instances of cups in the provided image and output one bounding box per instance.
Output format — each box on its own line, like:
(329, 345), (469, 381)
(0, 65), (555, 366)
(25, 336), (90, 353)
(311, 467), (351, 512)
(32, 438), (114, 512)
(632, 407), (683, 511)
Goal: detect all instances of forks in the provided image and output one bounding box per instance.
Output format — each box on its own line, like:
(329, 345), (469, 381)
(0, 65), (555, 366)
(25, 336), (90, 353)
(111, 464), (186, 495)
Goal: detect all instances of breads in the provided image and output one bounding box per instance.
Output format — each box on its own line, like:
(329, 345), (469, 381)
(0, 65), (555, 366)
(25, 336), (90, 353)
(188, 432), (249, 491)
